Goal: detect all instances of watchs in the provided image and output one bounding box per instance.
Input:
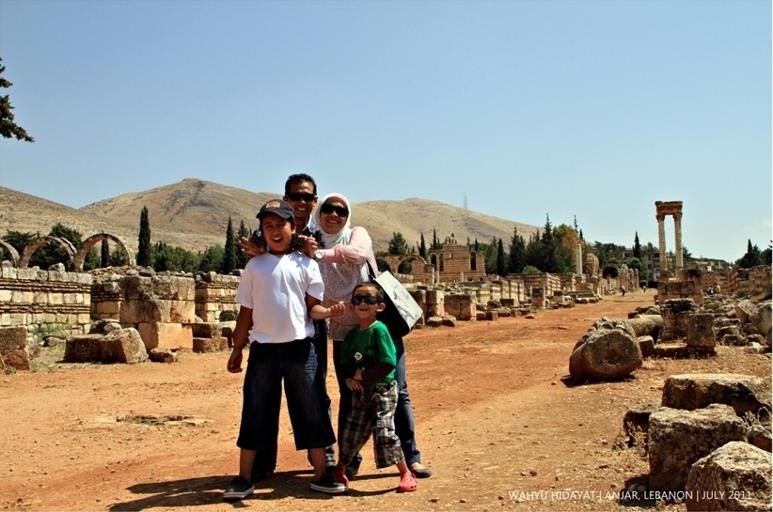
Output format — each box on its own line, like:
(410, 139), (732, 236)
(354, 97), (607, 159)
(313, 247), (324, 261)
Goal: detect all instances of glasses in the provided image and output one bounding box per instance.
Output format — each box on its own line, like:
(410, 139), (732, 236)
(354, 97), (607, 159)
(288, 192), (318, 203)
(350, 295), (379, 305)
(320, 203), (348, 217)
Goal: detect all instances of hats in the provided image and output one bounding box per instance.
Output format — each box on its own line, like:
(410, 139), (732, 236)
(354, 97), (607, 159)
(255, 198), (296, 222)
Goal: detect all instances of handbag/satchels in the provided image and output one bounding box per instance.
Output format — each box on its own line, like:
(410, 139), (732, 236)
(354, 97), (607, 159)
(364, 259), (424, 338)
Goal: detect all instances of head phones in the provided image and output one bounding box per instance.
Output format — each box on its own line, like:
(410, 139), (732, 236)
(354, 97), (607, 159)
(251, 228), (304, 251)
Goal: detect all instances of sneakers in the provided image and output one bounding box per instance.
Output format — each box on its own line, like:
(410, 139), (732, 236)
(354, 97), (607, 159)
(396, 461), (431, 492)
(223, 476), (256, 502)
(307, 447), (362, 496)
(251, 466), (275, 486)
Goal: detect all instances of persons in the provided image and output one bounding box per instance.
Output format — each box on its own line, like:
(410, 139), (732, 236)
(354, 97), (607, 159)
(713, 282), (720, 294)
(237, 191), (432, 483)
(620, 284), (625, 296)
(707, 284), (713, 295)
(333, 280), (419, 494)
(642, 284), (646, 294)
(220, 197), (348, 500)
(236, 172), (339, 484)
